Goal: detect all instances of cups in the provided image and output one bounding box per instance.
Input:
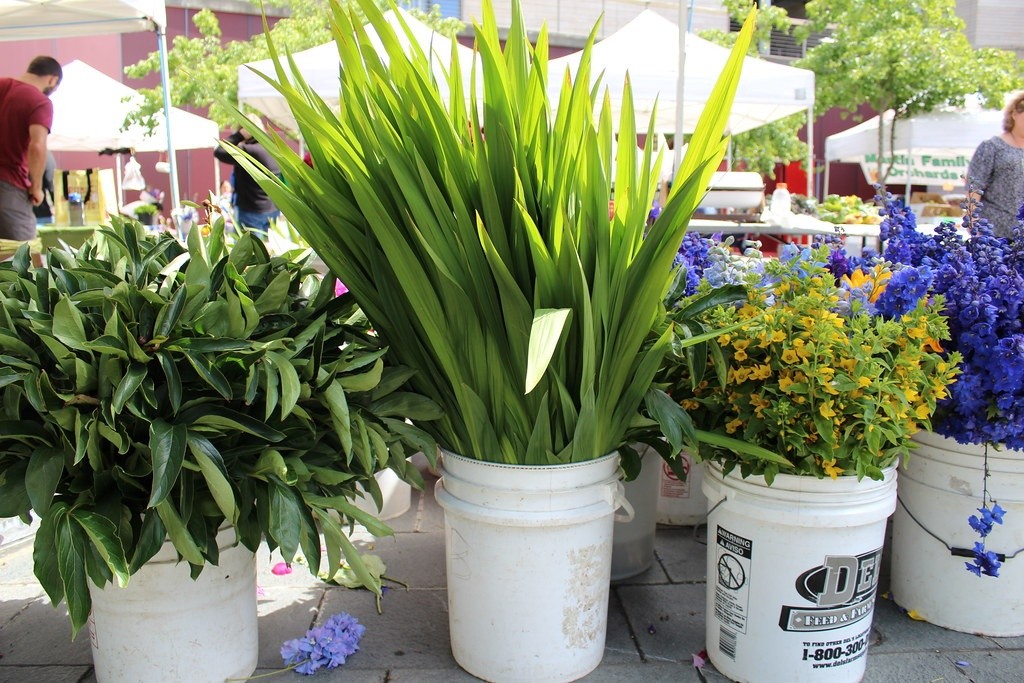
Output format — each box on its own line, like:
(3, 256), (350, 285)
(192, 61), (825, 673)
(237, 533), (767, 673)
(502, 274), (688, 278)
(67, 192), (83, 227)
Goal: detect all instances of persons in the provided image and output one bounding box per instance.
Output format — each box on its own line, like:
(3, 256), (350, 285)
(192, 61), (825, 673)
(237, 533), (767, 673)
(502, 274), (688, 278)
(214, 112), (285, 241)
(1, 56), (62, 241)
(965, 94), (1024, 239)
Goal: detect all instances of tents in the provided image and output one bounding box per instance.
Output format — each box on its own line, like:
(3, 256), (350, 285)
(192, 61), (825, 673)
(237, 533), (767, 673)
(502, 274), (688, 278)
(527, 7), (816, 204)
(0, 0), (184, 242)
(47, 59), (222, 226)
(823, 88), (1024, 210)
(238, 4), (485, 164)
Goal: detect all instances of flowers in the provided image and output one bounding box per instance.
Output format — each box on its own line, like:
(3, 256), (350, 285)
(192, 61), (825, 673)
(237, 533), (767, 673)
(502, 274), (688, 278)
(644, 179), (1024, 579)
(222, 610), (366, 682)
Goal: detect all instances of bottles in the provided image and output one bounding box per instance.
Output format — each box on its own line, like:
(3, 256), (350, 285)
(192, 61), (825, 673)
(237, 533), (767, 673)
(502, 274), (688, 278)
(771, 183), (792, 224)
